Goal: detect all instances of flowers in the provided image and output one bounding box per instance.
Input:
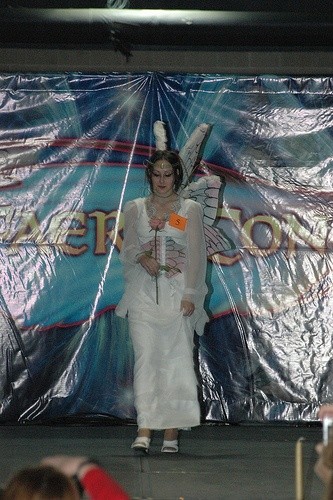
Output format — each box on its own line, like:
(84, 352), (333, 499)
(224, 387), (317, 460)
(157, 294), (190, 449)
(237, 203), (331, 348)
(145, 217), (181, 304)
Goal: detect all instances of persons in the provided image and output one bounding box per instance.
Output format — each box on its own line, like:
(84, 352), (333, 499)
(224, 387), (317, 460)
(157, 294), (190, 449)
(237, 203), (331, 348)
(115, 150), (210, 456)
(0, 454), (133, 500)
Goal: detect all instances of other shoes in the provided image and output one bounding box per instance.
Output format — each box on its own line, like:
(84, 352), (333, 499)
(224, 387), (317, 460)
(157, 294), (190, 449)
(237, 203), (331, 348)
(131, 437), (151, 454)
(161, 440), (178, 454)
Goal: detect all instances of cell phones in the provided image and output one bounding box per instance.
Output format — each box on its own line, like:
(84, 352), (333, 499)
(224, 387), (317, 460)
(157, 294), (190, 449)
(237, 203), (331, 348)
(323, 416), (333, 446)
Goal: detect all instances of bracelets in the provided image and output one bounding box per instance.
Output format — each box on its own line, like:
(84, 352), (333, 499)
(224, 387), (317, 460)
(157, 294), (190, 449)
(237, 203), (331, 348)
(75, 458), (101, 491)
